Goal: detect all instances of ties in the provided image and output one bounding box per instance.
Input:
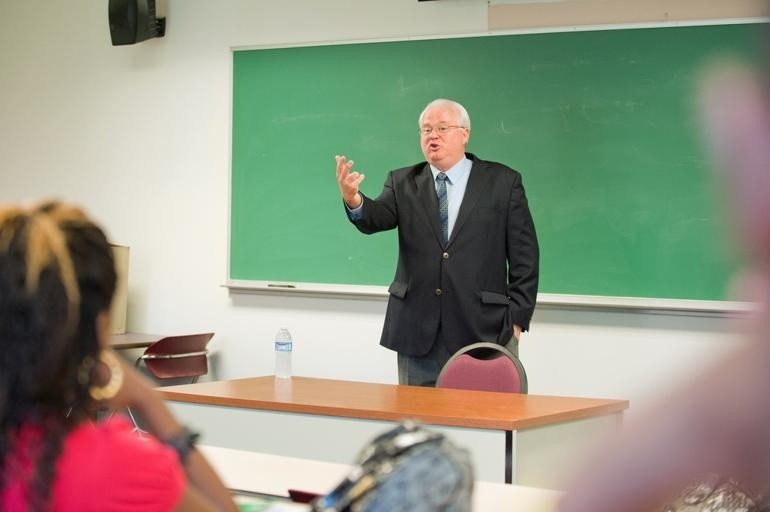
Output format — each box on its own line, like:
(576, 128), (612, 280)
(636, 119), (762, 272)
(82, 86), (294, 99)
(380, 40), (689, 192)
(436, 173), (449, 245)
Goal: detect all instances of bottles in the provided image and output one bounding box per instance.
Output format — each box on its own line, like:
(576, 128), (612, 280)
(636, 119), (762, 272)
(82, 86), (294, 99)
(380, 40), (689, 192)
(272, 325), (293, 379)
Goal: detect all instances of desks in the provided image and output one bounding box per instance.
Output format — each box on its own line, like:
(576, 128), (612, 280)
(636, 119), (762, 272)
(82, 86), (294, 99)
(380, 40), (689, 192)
(152, 374), (630, 486)
(197, 444), (568, 512)
(104, 332), (165, 348)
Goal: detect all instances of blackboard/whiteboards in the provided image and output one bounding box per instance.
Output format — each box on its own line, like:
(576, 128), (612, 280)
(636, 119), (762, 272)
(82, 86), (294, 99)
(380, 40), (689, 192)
(219, 17), (770, 315)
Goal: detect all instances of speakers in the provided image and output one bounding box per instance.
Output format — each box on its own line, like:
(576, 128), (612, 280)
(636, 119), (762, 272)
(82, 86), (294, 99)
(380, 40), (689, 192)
(108, 0), (166, 46)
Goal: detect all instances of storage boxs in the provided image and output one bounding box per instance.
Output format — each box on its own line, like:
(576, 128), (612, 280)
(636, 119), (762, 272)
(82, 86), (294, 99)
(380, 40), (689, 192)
(106, 242), (129, 336)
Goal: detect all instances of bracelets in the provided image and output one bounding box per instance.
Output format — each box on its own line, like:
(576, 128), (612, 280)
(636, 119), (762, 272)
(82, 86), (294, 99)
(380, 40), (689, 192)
(157, 424), (198, 463)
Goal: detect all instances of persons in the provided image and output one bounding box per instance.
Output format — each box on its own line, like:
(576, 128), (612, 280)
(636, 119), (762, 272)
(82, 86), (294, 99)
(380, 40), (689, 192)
(334, 96), (540, 386)
(0, 198), (237, 511)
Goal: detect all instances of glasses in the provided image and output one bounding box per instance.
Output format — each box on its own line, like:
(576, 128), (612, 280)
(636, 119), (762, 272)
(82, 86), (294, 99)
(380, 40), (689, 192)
(418, 126), (464, 135)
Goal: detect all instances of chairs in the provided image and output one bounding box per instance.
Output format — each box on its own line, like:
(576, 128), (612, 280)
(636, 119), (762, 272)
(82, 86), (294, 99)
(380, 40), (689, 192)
(435, 341), (528, 396)
(106, 333), (215, 440)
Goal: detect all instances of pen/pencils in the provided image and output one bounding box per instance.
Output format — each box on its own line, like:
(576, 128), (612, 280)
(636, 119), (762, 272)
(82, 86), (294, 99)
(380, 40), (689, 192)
(268, 285), (295, 287)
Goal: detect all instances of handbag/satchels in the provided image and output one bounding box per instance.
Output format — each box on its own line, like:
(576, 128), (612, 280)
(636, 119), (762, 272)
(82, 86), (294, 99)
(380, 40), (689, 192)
(346, 425), (473, 512)
